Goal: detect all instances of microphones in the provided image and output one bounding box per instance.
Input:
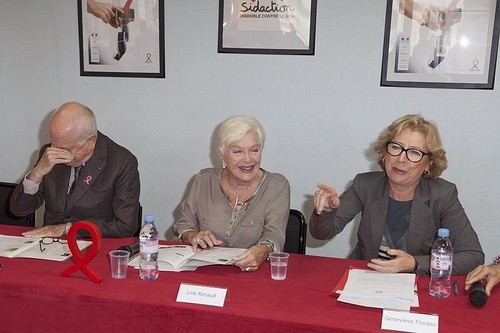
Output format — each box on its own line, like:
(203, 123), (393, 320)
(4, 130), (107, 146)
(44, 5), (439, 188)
(470, 282), (488, 307)
(115, 241), (139, 257)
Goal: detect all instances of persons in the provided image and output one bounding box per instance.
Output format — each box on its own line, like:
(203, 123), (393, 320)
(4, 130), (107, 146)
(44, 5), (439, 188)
(310, 114), (485, 277)
(465, 263), (500, 297)
(87, 0), (124, 29)
(399, 0), (445, 29)
(10, 102), (141, 239)
(173, 116), (290, 272)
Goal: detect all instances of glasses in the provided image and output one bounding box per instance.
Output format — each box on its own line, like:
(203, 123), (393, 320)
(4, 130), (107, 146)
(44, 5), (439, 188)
(386, 142), (431, 162)
(39, 235), (69, 252)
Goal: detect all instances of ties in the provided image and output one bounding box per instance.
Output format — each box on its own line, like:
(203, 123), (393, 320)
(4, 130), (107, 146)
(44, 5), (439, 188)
(66, 166), (82, 207)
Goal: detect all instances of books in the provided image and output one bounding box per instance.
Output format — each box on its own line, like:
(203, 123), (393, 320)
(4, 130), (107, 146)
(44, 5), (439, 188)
(0, 234), (92, 262)
(126, 244), (248, 272)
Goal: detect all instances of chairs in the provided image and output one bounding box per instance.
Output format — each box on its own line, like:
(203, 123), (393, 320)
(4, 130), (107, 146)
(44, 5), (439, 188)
(0, 182), (36, 227)
(283, 209), (307, 254)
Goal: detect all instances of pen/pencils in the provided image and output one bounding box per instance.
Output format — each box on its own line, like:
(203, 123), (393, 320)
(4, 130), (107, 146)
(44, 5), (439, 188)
(454, 281), (458, 296)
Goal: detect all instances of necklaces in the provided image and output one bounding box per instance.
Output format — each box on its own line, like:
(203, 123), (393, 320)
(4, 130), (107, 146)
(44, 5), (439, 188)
(227, 180), (254, 207)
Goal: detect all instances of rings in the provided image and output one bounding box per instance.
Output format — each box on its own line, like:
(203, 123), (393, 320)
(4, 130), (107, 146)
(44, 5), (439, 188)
(245, 267), (251, 272)
(316, 192), (318, 197)
(196, 238), (201, 241)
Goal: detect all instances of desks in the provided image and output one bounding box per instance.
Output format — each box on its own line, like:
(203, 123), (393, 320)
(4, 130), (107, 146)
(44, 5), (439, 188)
(0, 224), (500, 333)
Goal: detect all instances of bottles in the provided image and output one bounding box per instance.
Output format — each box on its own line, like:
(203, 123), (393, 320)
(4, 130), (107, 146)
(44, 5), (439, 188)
(429, 228), (453, 298)
(139, 215), (159, 280)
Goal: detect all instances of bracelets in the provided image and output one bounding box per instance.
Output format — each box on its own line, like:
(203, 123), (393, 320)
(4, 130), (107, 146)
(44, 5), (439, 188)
(66, 222), (72, 235)
(492, 256), (500, 264)
(259, 242), (275, 261)
(178, 229), (194, 237)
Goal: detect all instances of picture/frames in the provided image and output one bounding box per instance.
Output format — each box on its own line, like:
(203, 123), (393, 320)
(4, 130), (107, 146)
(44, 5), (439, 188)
(218, 0), (317, 56)
(78, 0), (165, 78)
(380, 0), (500, 89)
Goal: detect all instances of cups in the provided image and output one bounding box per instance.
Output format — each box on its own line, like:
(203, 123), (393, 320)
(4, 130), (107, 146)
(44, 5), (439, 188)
(109, 250), (130, 279)
(269, 252), (289, 280)
